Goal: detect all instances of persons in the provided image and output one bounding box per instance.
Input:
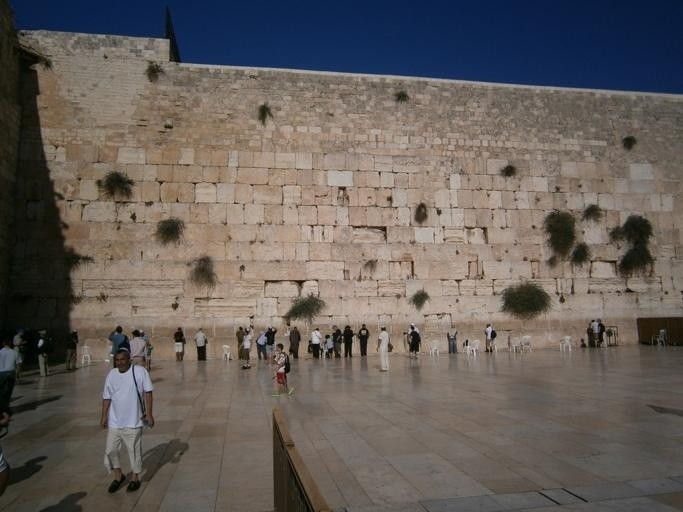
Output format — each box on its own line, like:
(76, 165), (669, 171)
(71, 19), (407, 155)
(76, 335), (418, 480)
(485, 323), (493, 352)
(587, 320), (606, 348)
(289, 327), (300, 359)
(174, 328), (185, 361)
(0, 340), (23, 418)
(109, 325), (152, 370)
(194, 328), (208, 361)
(13, 328), (79, 375)
(407, 322), (421, 359)
(0, 415), (10, 497)
(235, 324), (277, 369)
(100, 346), (156, 494)
(311, 324), (370, 360)
(447, 324), (458, 353)
(272, 342), (294, 397)
(376, 326), (389, 371)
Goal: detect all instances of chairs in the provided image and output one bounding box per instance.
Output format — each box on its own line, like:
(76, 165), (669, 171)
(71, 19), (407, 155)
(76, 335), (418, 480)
(522, 335), (536, 355)
(559, 335), (573, 352)
(509, 335), (523, 355)
(82, 345), (92, 365)
(651, 328), (666, 347)
(430, 339), (440, 357)
(462, 340), (480, 356)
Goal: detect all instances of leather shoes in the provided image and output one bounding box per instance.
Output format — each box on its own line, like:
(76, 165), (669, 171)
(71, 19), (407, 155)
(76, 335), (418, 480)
(127, 480), (141, 491)
(108, 473), (126, 493)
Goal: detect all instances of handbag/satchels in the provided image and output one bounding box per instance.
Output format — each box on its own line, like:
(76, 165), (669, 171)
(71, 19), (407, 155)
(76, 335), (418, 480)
(141, 415), (154, 432)
(491, 330), (496, 338)
